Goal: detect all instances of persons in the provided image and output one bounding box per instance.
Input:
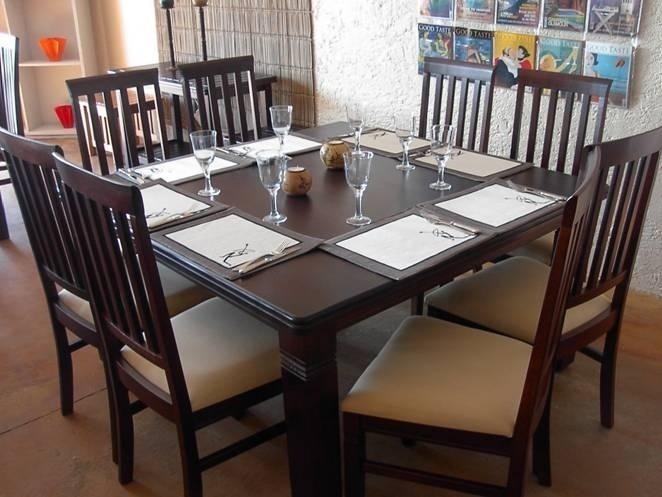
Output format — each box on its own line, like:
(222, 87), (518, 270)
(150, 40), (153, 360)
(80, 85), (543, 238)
(586, 52), (600, 77)
(420, 31), (433, 59)
(516, 46), (531, 69)
(494, 48), (518, 89)
(435, 32), (449, 58)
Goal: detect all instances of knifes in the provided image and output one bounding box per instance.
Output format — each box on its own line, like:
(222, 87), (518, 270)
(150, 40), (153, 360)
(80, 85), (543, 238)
(421, 210), (481, 234)
(120, 168), (144, 185)
(234, 244), (301, 271)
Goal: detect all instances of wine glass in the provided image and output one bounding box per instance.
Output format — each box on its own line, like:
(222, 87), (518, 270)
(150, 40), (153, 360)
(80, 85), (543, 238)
(346, 103), (366, 152)
(430, 124), (455, 190)
(191, 129), (221, 196)
(343, 151), (372, 226)
(256, 148), (287, 225)
(270, 104), (292, 150)
(393, 112), (416, 170)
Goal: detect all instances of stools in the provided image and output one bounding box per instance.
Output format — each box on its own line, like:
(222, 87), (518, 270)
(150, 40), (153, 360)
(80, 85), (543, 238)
(78, 89), (163, 156)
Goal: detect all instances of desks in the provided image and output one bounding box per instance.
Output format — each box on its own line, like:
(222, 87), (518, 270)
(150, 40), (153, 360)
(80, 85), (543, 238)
(106, 61), (277, 144)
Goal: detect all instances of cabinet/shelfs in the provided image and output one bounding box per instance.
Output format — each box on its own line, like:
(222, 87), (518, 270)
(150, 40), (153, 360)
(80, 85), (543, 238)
(0, 0), (102, 142)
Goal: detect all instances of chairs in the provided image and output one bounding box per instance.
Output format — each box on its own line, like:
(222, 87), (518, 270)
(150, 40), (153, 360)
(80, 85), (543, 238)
(0, 47), (9, 240)
(50, 152), (285, 497)
(177, 55), (261, 153)
(339, 144), (600, 496)
(64, 67), (169, 176)
(0, 130), (215, 464)
(416, 56), (495, 155)
(488, 67), (612, 263)
(0, 32), (23, 186)
(424, 125), (661, 428)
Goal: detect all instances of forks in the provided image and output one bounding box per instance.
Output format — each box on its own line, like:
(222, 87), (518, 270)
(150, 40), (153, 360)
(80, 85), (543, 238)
(149, 202), (199, 228)
(506, 180), (564, 200)
(239, 238), (291, 273)
